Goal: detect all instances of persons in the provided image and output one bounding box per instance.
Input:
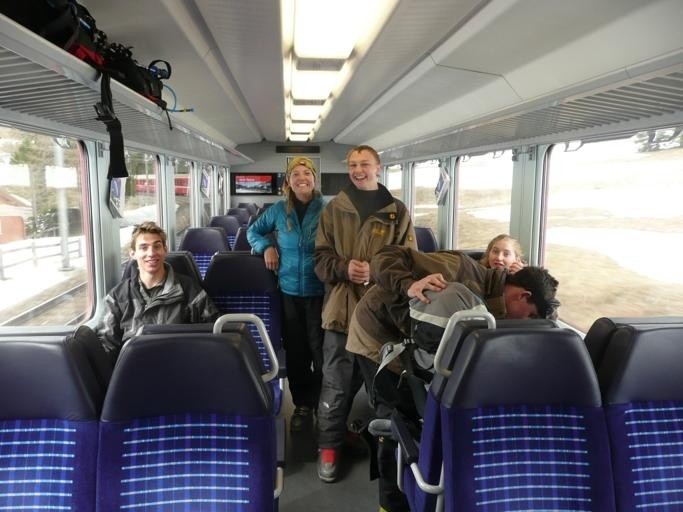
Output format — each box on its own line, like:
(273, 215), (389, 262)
(101, 221), (220, 357)
(347, 246), (559, 512)
(246, 156), (329, 433)
(477, 234), (526, 276)
(312, 145), (418, 482)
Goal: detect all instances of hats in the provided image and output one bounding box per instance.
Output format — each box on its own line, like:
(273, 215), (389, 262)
(287, 157), (317, 179)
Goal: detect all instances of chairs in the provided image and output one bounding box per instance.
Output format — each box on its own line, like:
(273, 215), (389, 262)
(461, 248), (486, 261)
(1, 202), (295, 512)
(412, 225), (439, 253)
(391, 310), (682, 512)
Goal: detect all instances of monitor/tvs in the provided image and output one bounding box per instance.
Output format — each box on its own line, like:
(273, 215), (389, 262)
(321, 172), (349, 196)
(230, 172), (277, 195)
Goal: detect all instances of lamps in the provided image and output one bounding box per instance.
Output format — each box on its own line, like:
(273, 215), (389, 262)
(287, 0), (369, 141)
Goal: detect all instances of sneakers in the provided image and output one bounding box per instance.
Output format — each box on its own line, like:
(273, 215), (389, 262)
(290, 406), (319, 435)
(316, 446), (341, 483)
(345, 431), (367, 448)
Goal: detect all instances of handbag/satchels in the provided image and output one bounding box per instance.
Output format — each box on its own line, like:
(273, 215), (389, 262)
(0, 0), (162, 99)
(409, 282), (488, 391)
